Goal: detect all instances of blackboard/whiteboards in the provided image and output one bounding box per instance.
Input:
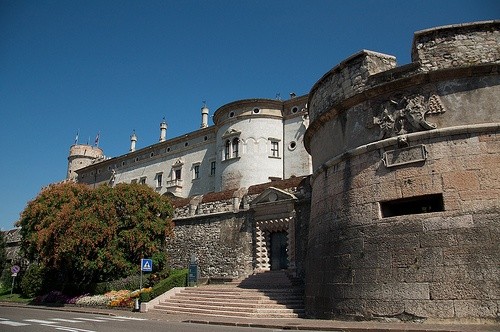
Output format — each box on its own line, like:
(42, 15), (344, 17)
(188, 264), (198, 283)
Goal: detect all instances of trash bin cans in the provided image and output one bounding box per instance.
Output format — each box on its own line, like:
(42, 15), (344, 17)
(134, 297), (140, 310)
